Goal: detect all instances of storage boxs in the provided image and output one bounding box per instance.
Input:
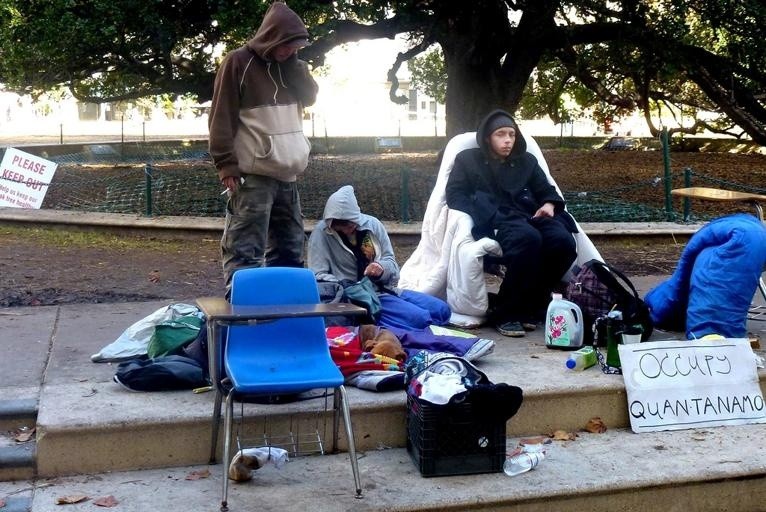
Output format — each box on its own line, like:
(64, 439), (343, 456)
(406, 389), (507, 478)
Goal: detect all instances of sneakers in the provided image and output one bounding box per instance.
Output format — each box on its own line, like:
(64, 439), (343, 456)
(496, 320), (536, 337)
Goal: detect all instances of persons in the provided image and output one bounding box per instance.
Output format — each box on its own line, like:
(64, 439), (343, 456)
(307, 184), (451, 326)
(209, 2), (320, 302)
(446, 110), (576, 336)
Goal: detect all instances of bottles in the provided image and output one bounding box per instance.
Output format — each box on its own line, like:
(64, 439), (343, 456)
(606, 311), (624, 366)
(504, 453), (545, 477)
(567, 345), (597, 372)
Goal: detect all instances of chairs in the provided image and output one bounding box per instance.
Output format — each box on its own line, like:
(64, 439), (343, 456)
(209, 267), (364, 512)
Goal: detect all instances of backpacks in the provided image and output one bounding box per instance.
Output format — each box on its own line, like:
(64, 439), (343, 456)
(567, 259), (653, 343)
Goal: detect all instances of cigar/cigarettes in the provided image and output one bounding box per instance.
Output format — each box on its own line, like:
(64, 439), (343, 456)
(219, 187), (231, 194)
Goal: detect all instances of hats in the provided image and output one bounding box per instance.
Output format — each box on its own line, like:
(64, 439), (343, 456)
(285, 37), (315, 48)
(489, 114), (515, 135)
